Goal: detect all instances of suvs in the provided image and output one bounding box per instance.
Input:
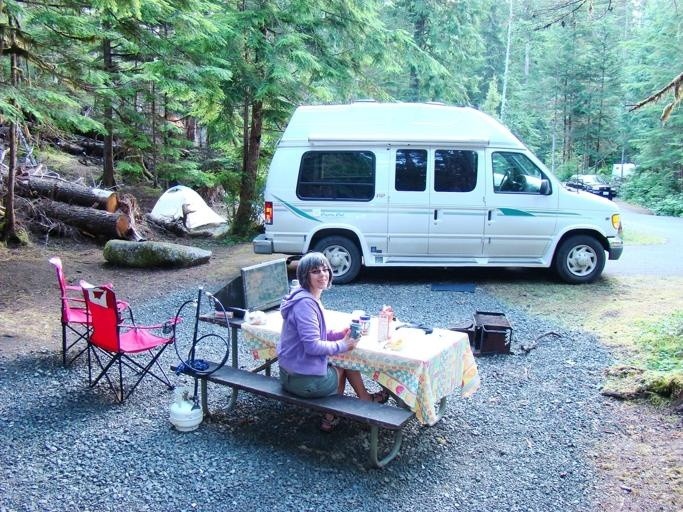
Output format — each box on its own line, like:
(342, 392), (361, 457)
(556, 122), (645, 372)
(568, 170), (619, 205)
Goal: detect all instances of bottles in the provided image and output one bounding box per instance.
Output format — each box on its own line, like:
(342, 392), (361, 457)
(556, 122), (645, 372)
(349, 315), (370, 339)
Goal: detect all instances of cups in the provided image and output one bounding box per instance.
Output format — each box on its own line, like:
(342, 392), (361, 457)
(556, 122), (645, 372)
(377, 310), (393, 342)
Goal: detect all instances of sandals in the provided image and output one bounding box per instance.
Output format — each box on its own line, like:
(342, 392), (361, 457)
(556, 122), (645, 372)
(368, 389), (391, 404)
(320, 416), (342, 433)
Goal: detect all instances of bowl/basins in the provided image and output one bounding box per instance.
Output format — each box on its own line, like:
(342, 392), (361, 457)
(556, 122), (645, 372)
(169, 411), (203, 432)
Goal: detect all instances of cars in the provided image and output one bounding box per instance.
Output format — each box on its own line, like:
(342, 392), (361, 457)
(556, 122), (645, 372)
(253, 104), (622, 285)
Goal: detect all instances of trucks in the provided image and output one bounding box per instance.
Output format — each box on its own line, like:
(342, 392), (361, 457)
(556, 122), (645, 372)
(610, 161), (636, 183)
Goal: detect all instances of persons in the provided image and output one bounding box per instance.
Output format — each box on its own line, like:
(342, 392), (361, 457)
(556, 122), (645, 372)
(276, 252), (389, 434)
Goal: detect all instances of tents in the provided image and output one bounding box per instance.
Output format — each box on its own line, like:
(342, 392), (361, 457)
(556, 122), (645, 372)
(150, 185), (226, 228)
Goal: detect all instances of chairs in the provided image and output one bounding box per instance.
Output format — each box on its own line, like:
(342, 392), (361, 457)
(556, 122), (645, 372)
(79, 280), (183, 402)
(48, 257), (135, 368)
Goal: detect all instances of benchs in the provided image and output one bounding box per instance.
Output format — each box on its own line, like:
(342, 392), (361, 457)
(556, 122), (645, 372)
(172, 358), (415, 467)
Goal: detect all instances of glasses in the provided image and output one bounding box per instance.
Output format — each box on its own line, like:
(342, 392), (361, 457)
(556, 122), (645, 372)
(308, 267), (329, 275)
